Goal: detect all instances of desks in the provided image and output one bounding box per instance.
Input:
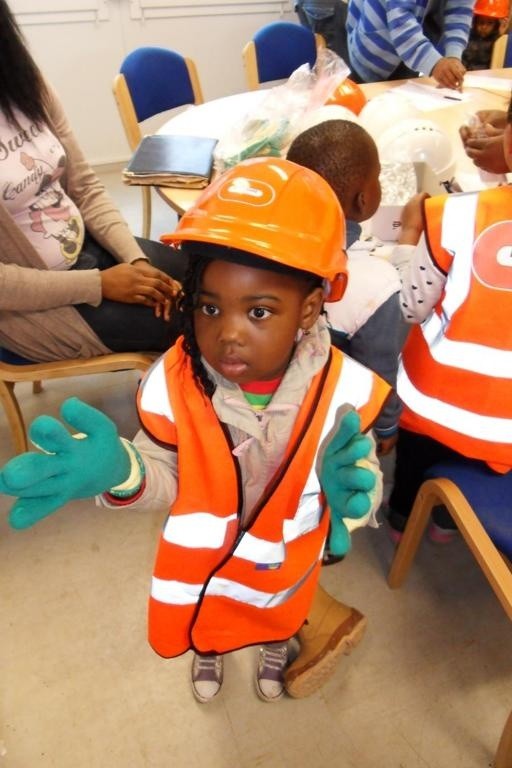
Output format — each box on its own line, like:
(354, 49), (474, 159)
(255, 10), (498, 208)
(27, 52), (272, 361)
(152, 65), (512, 250)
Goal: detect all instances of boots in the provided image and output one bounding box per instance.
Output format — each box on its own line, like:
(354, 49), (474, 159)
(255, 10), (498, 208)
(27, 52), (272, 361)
(283, 586), (368, 697)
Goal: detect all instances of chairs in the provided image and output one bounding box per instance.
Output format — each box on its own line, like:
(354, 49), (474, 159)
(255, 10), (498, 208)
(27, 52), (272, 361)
(241, 20), (327, 94)
(111, 45), (206, 241)
(385, 457), (511, 768)
(1, 343), (162, 457)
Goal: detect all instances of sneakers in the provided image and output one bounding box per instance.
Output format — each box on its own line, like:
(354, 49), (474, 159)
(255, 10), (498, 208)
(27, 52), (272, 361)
(256, 641), (290, 704)
(193, 651), (226, 701)
(387, 521), (458, 546)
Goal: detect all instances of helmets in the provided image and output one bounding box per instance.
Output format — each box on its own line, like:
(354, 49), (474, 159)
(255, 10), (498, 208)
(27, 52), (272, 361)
(160, 156), (349, 280)
(472, 0), (512, 19)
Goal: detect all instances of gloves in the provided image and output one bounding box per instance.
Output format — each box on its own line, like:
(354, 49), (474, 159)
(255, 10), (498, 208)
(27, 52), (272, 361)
(1, 395), (148, 527)
(323, 411), (375, 557)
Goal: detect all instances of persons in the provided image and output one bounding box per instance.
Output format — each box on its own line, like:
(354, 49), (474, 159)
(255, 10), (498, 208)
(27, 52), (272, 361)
(285, 121), (404, 458)
(1, 0), (189, 367)
(281, 1), (511, 93)
(0, 154), (391, 711)
(386, 95), (512, 550)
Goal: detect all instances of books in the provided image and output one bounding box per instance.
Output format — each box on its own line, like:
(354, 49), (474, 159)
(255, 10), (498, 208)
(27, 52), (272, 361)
(122, 133), (220, 192)
